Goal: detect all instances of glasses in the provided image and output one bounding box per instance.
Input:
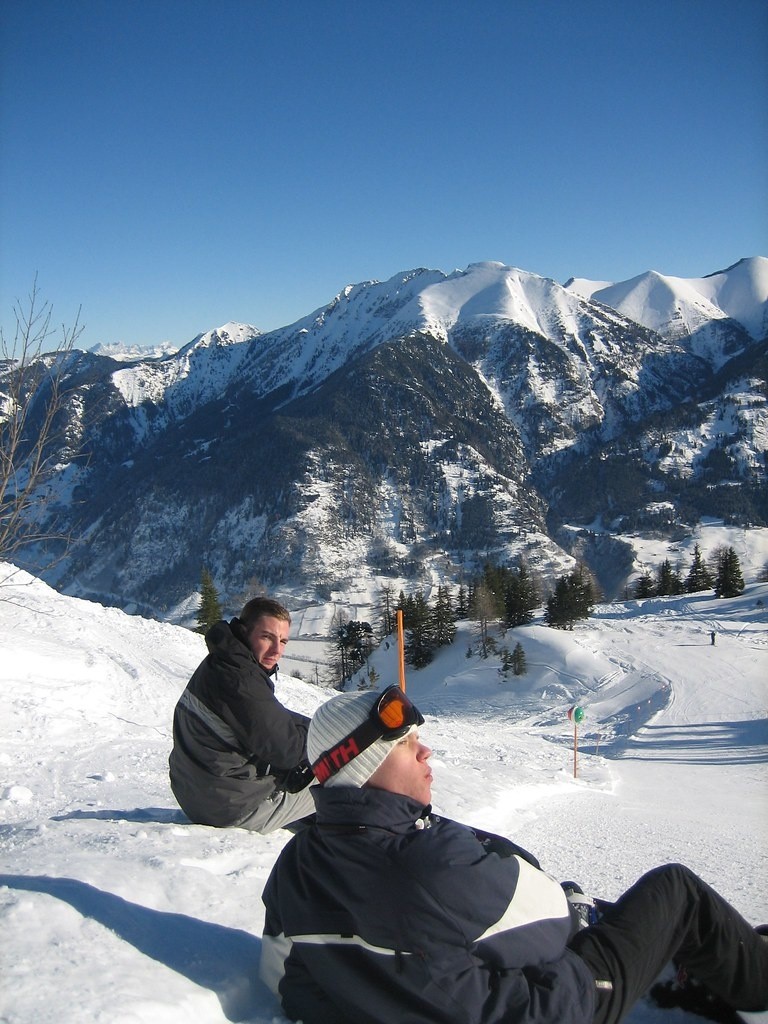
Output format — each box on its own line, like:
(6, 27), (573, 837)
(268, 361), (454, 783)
(309, 682), (425, 784)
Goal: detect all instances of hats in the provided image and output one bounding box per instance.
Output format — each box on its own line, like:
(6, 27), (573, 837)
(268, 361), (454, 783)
(307, 690), (418, 788)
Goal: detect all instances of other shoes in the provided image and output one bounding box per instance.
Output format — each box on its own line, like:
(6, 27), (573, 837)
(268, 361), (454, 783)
(560, 882), (589, 932)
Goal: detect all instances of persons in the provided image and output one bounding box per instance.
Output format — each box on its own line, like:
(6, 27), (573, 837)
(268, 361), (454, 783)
(711, 631), (715, 645)
(262, 684), (768, 1024)
(168, 596), (313, 835)
